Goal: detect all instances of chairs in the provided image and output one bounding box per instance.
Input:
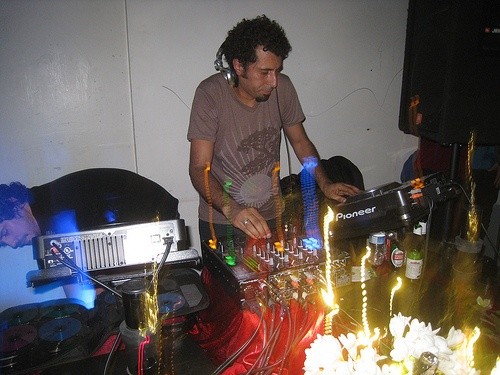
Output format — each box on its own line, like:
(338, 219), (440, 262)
(27, 167), (186, 299)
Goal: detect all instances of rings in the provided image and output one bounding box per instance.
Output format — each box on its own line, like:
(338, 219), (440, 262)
(243, 219), (249, 224)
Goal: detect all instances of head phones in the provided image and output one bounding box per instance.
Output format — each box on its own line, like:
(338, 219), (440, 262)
(214, 41), (240, 89)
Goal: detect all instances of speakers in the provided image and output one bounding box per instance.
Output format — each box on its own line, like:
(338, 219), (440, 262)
(397, 0), (500, 146)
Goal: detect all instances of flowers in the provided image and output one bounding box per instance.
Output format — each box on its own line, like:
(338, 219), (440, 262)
(302, 312), (480, 375)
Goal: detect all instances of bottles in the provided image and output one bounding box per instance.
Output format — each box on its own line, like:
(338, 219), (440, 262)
(365, 216), (426, 280)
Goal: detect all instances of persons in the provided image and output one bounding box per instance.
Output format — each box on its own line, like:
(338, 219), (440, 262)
(460, 145), (500, 241)
(0, 167), (181, 300)
(186, 14), (361, 259)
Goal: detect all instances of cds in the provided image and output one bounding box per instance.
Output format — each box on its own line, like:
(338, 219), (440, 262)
(158, 279), (185, 314)
(0, 301), (82, 352)
